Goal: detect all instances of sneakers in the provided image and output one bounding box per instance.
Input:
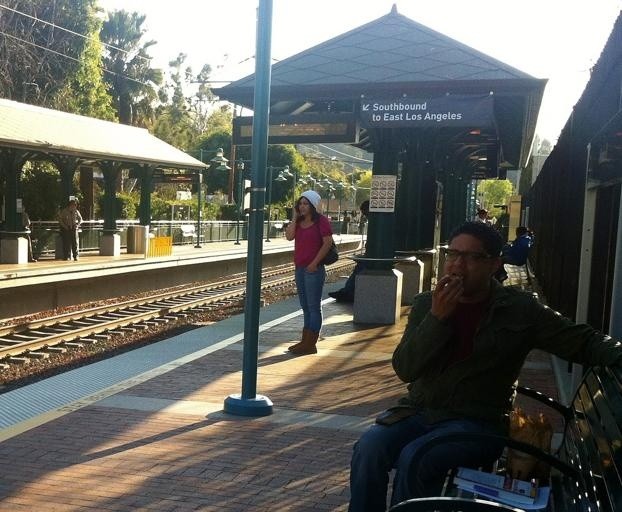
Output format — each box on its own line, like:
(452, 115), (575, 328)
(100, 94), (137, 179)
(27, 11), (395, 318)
(500, 274), (508, 281)
(328, 291), (354, 302)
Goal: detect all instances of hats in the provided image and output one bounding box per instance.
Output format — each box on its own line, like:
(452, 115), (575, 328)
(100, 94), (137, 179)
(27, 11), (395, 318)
(301, 190), (322, 208)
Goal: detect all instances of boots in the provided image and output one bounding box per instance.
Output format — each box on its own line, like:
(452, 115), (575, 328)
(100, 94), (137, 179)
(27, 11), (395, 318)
(288, 327), (319, 353)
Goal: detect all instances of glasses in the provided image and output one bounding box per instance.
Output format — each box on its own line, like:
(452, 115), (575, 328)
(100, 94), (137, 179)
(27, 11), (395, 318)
(444, 249), (500, 264)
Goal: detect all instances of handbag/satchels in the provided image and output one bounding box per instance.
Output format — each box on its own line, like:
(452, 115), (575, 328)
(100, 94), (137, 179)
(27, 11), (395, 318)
(323, 237), (338, 265)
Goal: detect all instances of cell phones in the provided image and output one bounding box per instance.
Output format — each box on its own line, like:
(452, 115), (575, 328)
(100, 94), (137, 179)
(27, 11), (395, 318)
(295, 204), (299, 213)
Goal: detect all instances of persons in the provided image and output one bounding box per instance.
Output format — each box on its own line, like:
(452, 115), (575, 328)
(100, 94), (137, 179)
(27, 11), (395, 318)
(474, 209), (488, 224)
(285, 191), (335, 354)
(22, 205), (37, 263)
(328, 201), (368, 300)
(347, 223), (622, 509)
(494, 225), (529, 283)
(57, 200), (85, 262)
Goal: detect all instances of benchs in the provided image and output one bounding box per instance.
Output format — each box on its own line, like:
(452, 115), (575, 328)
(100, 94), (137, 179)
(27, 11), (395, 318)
(181, 225), (205, 246)
(387, 356), (622, 511)
(500, 240), (535, 285)
